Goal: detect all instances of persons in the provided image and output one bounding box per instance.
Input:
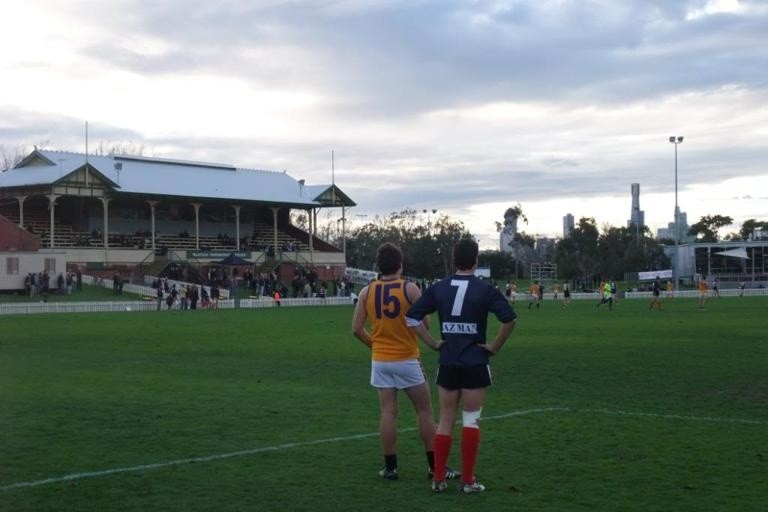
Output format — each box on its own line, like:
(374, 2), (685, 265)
(416, 278), (434, 290)
(528, 280), (544, 309)
(199, 230), (300, 263)
(112, 271), (123, 295)
(243, 267), (288, 306)
(699, 276), (709, 306)
(598, 279), (616, 308)
(25, 270), (51, 302)
(152, 265), (238, 310)
(334, 275), (358, 305)
(553, 281), (571, 304)
(57, 271), (82, 294)
(627, 275), (673, 310)
(404, 239), (517, 493)
(494, 280), (519, 304)
(741, 280), (745, 297)
(713, 278), (720, 297)
(293, 269), (326, 298)
(352, 243), (462, 481)
(29, 228), (189, 260)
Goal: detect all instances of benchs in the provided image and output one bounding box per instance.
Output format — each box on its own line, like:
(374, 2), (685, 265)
(0, 207), (318, 252)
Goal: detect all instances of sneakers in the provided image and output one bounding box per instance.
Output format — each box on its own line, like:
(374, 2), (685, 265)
(379, 465), (485, 493)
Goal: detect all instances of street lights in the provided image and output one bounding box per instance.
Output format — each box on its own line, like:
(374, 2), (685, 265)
(668, 134), (684, 291)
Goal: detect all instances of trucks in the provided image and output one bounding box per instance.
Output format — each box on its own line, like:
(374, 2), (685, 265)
(473, 267), (490, 281)
